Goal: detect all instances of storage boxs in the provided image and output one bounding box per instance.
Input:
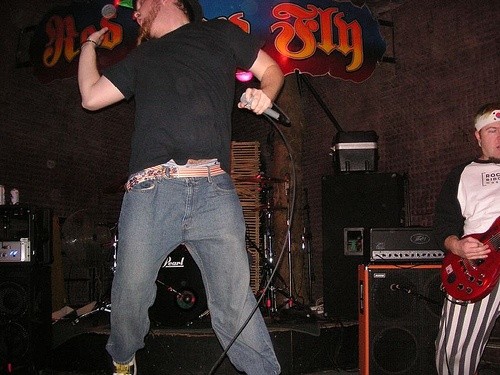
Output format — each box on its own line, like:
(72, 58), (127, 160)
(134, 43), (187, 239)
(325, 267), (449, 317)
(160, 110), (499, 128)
(330, 131), (379, 172)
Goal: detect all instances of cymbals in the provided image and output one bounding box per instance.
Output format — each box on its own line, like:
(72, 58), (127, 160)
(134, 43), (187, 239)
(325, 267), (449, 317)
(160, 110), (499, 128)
(234, 176), (285, 183)
(251, 205), (287, 212)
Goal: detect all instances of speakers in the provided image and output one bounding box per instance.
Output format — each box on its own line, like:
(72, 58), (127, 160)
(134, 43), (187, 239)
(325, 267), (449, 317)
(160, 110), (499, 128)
(0, 261), (65, 375)
(146, 245), (208, 328)
(355, 263), (448, 375)
(320, 171), (404, 326)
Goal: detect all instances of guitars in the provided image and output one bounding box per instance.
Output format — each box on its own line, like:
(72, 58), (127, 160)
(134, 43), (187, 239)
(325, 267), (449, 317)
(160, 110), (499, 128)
(441, 216), (500, 305)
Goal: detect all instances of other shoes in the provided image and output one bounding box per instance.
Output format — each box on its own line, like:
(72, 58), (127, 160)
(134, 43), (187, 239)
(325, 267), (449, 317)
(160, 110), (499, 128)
(112, 356), (137, 375)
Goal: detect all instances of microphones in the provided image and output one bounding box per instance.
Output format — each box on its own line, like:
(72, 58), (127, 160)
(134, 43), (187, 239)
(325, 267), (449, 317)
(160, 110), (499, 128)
(240, 93), (293, 125)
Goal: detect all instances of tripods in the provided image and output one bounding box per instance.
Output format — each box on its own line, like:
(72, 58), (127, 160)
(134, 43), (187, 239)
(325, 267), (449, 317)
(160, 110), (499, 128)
(71, 241), (110, 326)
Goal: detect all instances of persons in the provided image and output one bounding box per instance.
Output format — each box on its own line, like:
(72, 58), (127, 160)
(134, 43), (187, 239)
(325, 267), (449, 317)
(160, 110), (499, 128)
(76, 0), (285, 375)
(434, 102), (500, 375)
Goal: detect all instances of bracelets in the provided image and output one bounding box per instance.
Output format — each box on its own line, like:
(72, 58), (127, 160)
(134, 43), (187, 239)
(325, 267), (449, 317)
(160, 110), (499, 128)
(81, 40), (97, 47)
